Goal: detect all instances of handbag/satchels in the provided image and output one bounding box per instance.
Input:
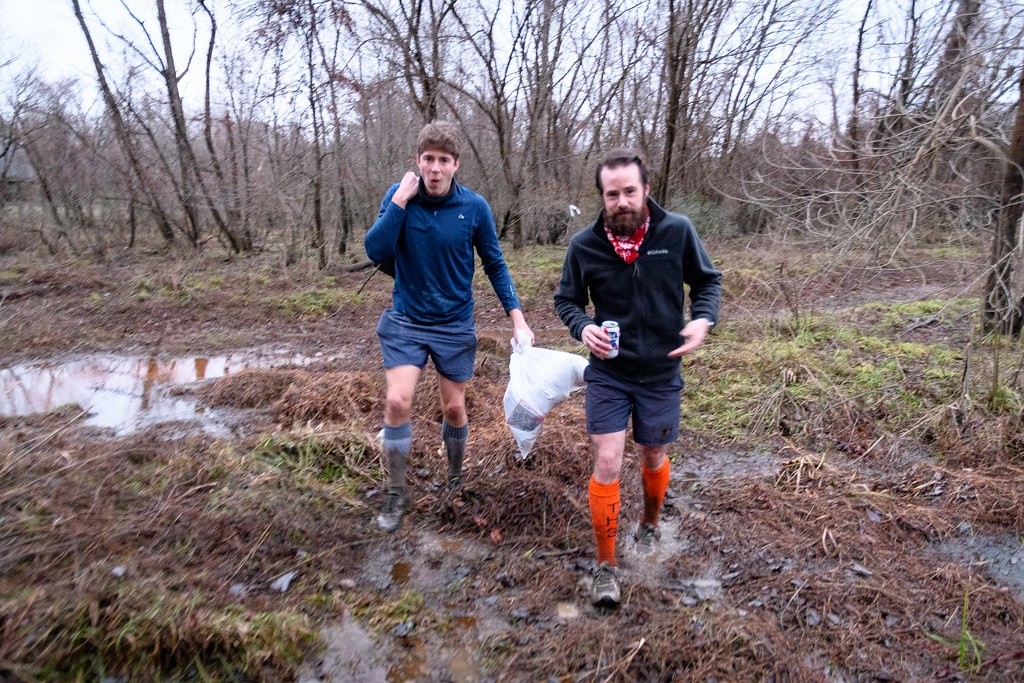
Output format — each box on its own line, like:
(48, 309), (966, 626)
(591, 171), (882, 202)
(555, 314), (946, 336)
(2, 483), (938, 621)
(503, 331), (590, 459)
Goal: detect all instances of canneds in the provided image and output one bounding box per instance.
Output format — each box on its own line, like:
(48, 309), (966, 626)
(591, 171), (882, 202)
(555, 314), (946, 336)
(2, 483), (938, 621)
(601, 321), (620, 360)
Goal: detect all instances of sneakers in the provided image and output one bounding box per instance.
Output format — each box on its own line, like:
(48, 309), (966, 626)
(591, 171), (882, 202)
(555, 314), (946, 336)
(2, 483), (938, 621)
(586, 562), (623, 605)
(445, 472), (464, 490)
(634, 523), (662, 558)
(376, 486), (415, 533)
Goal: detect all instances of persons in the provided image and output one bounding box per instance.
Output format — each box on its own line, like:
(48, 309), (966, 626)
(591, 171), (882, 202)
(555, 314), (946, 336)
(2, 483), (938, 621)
(553, 149), (723, 605)
(364, 120), (535, 532)
